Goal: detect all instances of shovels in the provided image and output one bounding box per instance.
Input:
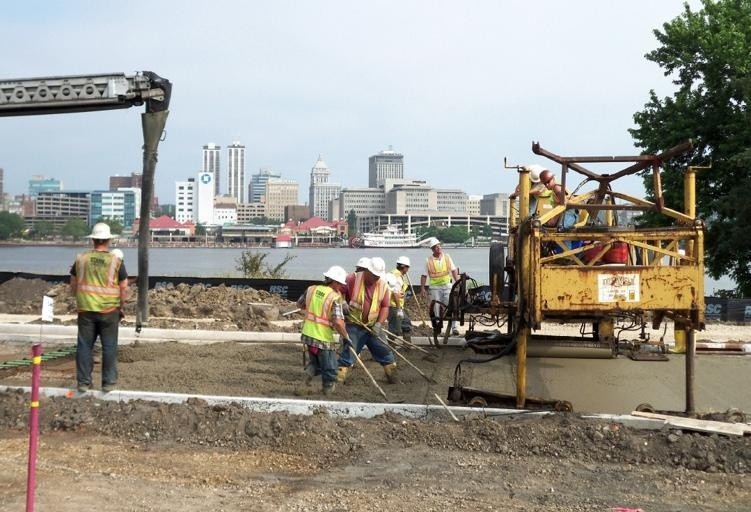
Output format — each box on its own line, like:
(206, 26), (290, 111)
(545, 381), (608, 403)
(351, 315), (438, 384)
(406, 273), (436, 350)
(383, 329), (439, 362)
(347, 345), (406, 403)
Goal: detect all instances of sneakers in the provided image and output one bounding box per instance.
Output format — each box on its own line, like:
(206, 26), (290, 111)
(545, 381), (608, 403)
(102, 383), (116, 393)
(78, 381), (94, 392)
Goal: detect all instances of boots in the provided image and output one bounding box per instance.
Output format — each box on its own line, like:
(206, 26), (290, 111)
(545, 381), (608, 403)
(336, 365), (349, 382)
(294, 365), (316, 395)
(402, 331), (412, 350)
(382, 361), (406, 384)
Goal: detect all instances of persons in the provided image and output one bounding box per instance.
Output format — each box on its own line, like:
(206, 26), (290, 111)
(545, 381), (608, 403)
(420, 238), (459, 335)
(69, 223), (128, 392)
(540, 170), (578, 250)
(296, 266), (354, 396)
(511, 165), (552, 217)
(387, 256), (412, 352)
(355, 257), (370, 273)
(337, 257), (405, 384)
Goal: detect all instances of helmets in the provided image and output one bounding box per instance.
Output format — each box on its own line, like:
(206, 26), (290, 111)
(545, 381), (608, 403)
(539, 169), (554, 183)
(323, 265), (348, 285)
(111, 247), (125, 261)
(429, 238), (441, 248)
(530, 167), (541, 183)
(86, 223), (118, 240)
(367, 257), (387, 277)
(396, 255), (411, 268)
(355, 257), (370, 269)
